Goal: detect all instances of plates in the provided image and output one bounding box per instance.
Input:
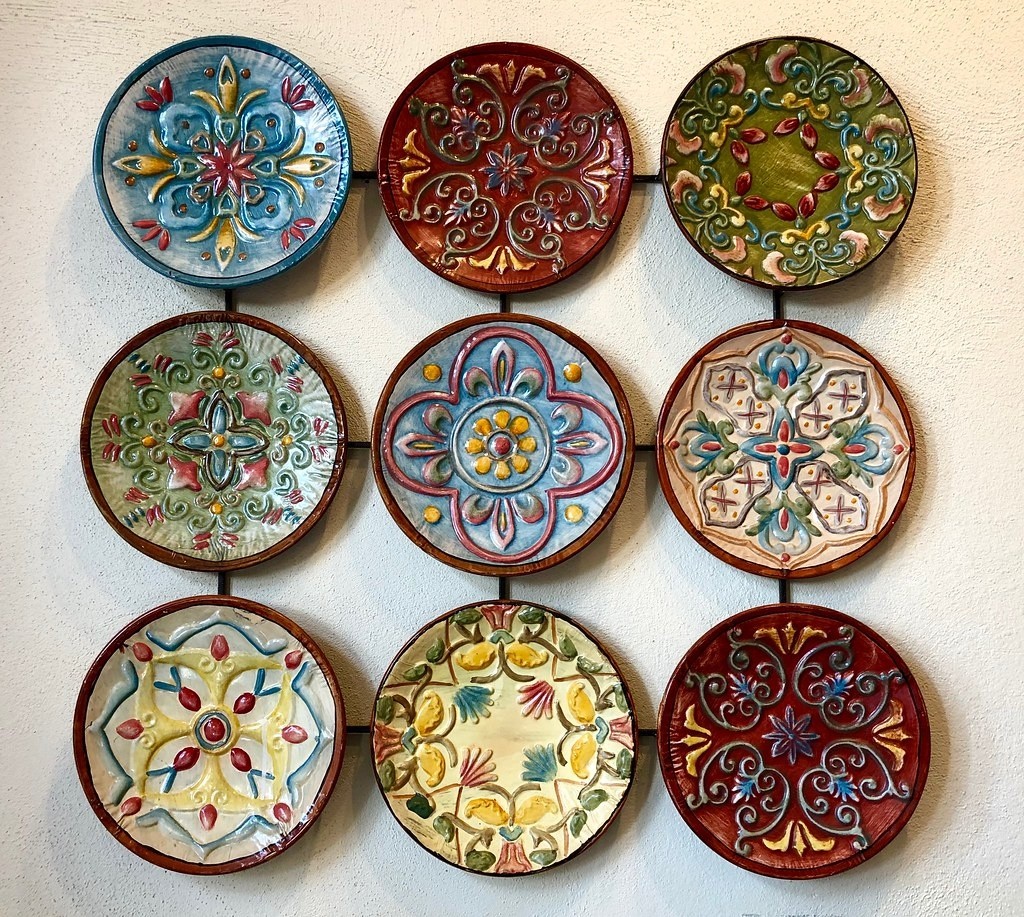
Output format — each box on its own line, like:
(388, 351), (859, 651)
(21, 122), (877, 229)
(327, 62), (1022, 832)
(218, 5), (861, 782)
(368, 314), (636, 577)
(78, 310), (348, 572)
(660, 35), (918, 291)
(654, 321), (917, 578)
(368, 599), (641, 878)
(655, 602), (931, 881)
(378, 41), (633, 295)
(72, 595), (347, 877)
(92, 34), (352, 288)
